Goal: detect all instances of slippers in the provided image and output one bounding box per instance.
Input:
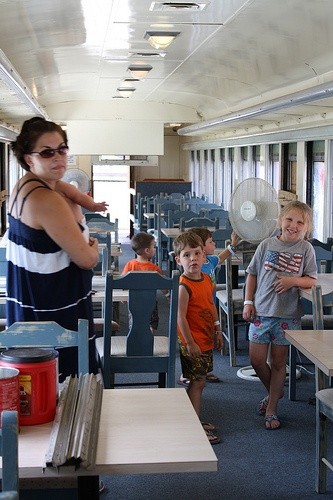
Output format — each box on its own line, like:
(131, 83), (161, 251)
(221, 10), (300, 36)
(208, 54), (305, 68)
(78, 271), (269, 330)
(264, 409), (281, 430)
(177, 377), (190, 387)
(256, 389), (284, 416)
(206, 373), (219, 383)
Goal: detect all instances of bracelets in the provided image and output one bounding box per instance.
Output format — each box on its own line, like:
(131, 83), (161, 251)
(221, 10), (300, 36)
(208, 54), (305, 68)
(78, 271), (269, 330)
(244, 301), (253, 305)
(164, 293), (169, 297)
(227, 244), (235, 253)
(215, 330), (221, 335)
(214, 320), (220, 326)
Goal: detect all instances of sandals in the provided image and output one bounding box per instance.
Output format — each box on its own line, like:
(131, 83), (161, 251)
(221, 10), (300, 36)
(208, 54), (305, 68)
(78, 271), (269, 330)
(207, 432), (221, 445)
(201, 423), (216, 432)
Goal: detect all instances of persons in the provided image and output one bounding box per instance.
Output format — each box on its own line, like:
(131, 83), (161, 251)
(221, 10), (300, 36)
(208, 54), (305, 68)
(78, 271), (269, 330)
(243, 200), (317, 430)
(188, 226), (238, 304)
(5, 116), (110, 384)
(120, 232), (170, 335)
(174, 232), (224, 444)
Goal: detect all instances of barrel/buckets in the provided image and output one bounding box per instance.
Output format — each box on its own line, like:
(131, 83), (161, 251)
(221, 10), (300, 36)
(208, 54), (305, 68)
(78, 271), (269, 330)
(0, 347), (59, 424)
(0, 366), (21, 434)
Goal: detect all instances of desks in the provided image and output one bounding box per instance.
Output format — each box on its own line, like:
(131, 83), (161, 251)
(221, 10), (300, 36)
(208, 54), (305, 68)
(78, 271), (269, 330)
(143, 213), (164, 233)
(160, 227), (222, 276)
(296, 273), (333, 306)
(284, 329), (333, 414)
(0, 387), (218, 500)
(97, 243), (122, 273)
(92, 288), (158, 326)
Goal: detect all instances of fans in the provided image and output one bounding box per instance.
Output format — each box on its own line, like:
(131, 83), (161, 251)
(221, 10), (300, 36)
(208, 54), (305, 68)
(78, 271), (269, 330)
(228, 177), (281, 245)
(60, 168), (90, 194)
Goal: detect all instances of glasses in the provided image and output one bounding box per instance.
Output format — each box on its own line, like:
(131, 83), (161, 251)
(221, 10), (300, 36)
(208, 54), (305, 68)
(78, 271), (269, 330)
(30, 145), (69, 159)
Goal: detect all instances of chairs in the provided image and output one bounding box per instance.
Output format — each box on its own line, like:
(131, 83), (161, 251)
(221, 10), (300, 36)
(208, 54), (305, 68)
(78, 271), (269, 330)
(0, 191), (333, 500)
